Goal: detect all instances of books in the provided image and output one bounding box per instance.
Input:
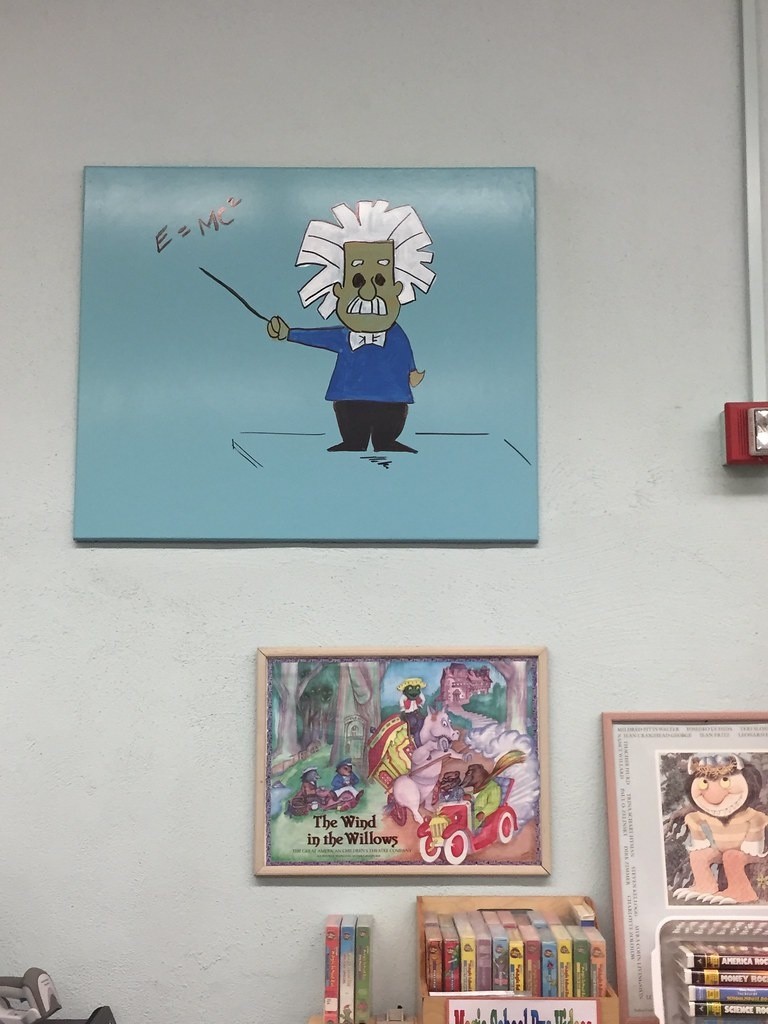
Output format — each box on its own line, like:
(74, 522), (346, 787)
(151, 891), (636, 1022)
(670, 946), (768, 1024)
(424, 901), (608, 997)
(324, 914), (372, 1024)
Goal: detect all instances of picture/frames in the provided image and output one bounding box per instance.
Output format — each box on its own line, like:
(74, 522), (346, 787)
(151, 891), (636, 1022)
(602, 711), (768, 1024)
(254, 647), (551, 877)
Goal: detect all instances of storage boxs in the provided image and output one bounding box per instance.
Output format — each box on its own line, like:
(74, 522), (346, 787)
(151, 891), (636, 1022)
(416, 895), (619, 1024)
